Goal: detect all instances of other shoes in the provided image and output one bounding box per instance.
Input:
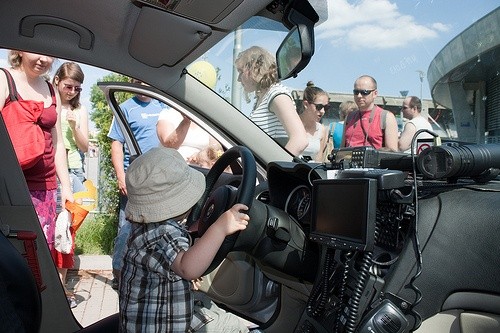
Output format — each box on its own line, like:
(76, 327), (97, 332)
(65, 288), (78, 308)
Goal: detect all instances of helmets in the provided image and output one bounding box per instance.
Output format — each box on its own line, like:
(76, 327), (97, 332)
(189, 60), (217, 89)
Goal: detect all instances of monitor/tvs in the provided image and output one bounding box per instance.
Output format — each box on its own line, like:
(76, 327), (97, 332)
(311, 177), (378, 251)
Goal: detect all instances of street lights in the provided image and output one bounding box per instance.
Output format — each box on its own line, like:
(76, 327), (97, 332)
(419, 69), (426, 101)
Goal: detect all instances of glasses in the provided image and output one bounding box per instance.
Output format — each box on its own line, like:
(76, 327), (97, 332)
(311, 102), (328, 111)
(352, 89), (375, 95)
(65, 84), (82, 92)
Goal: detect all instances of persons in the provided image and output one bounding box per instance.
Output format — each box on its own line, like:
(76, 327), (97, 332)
(297, 81), (330, 164)
(340, 75), (399, 153)
(398, 96), (434, 155)
(236, 46), (309, 186)
(156, 59), (217, 226)
(106, 79), (169, 290)
(118, 147), (262, 333)
(0, 50), (89, 309)
(320, 100), (358, 162)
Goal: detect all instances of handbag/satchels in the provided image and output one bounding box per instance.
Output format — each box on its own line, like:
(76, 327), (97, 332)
(322, 122), (334, 162)
(0, 67), (45, 170)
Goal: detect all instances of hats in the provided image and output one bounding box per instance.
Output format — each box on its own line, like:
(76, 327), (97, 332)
(125, 148), (207, 223)
(54, 210), (73, 255)
